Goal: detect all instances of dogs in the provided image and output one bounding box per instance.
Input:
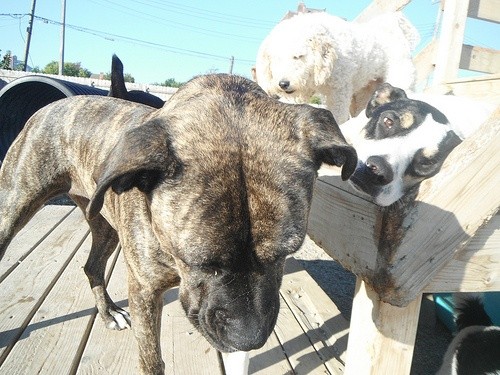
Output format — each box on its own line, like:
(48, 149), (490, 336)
(347, 82), (500, 212)
(0, 72), (358, 375)
(255, 4), (421, 125)
